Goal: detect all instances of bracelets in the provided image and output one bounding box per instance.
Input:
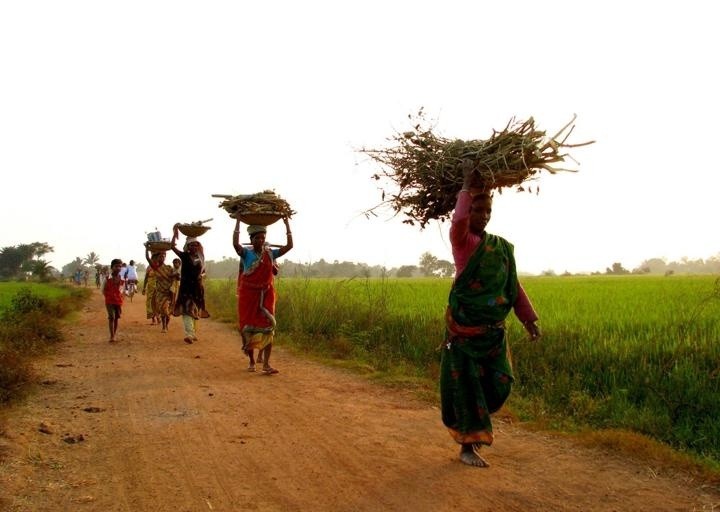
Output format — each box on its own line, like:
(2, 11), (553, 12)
(459, 189), (471, 194)
(234, 229), (241, 234)
(287, 232), (293, 237)
(143, 288), (146, 293)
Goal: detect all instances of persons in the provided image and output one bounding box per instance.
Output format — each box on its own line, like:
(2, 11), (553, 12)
(141, 253), (162, 326)
(170, 227), (206, 344)
(73, 259), (140, 296)
(236, 259), (280, 365)
(232, 208), (294, 375)
(170, 257), (181, 315)
(439, 158), (542, 469)
(146, 244), (174, 334)
(100, 259), (125, 343)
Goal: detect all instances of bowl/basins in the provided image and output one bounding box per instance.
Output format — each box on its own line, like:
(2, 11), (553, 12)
(143, 242), (176, 251)
(230, 212), (284, 225)
(147, 231), (161, 241)
(176, 226), (211, 236)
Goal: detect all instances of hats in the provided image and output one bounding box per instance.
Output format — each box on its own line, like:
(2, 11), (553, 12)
(247, 224), (267, 235)
(455, 187), (492, 202)
(186, 237), (199, 244)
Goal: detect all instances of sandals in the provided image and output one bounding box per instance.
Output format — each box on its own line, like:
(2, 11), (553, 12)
(247, 362), (257, 372)
(262, 367), (279, 375)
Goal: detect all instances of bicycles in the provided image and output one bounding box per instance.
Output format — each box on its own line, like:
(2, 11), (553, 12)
(128, 283), (136, 303)
